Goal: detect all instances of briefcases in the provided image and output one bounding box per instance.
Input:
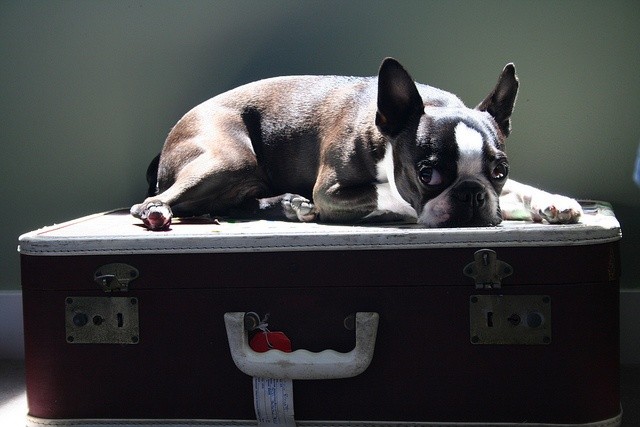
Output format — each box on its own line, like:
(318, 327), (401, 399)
(17, 198), (623, 427)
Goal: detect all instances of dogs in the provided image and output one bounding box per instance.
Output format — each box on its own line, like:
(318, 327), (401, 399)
(103, 57), (584, 228)
